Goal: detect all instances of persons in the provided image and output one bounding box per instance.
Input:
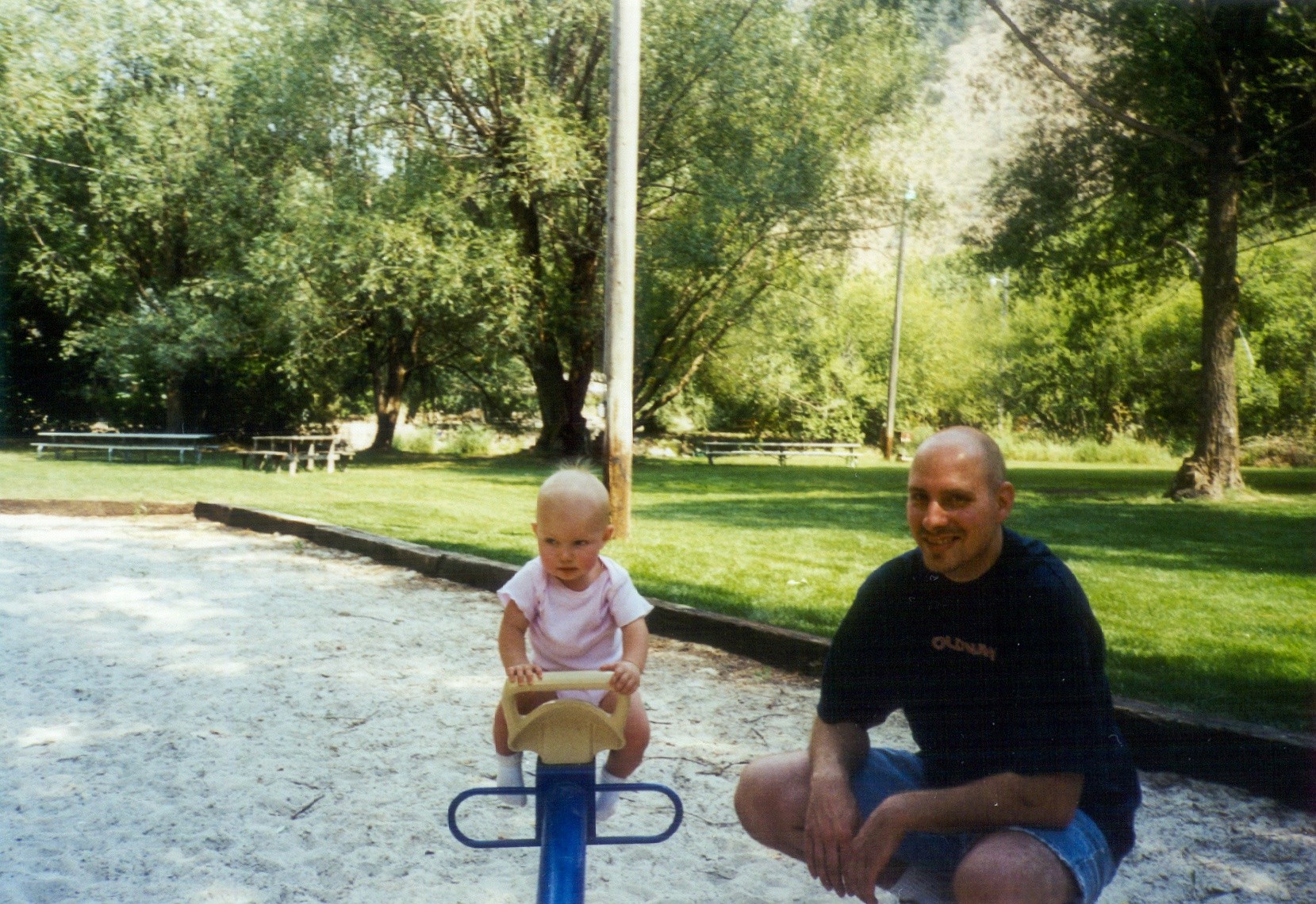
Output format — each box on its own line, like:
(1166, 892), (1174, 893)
(734, 425), (1142, 904)
(494, 468), (654, 822)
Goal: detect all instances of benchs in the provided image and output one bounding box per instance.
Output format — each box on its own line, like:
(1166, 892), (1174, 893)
(238, 449), (355, 471)
(703, 450), (861, 465)
(30, 440), (218, 464)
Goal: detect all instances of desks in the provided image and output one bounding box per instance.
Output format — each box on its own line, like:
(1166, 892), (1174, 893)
(702, 442), (862, 466)
(37, 432), (216, 463)
(250, 434), (340, 475)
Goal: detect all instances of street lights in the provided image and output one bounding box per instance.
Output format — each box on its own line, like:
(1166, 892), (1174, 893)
(882, 189), (919, 458)
